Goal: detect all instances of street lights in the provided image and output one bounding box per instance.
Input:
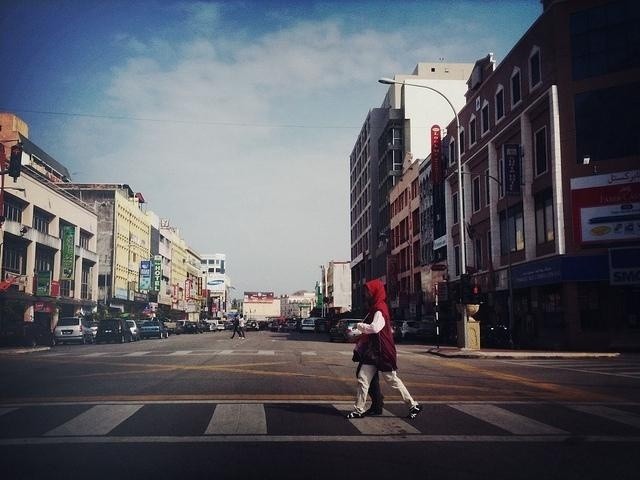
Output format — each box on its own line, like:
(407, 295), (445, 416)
(378, 80), (467, 274)
(454, 170), (514, 336)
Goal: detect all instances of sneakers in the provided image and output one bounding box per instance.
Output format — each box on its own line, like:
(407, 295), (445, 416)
(408, 405), (421, 420)
(345, 412), (362, 419)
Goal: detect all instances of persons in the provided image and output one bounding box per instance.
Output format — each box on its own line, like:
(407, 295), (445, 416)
(293, 320), (304, 337)
(311, 320), (322, 335)
(355, 295), (385, 418)
(342, 278), (423, 420)
(230, 309), (247, 340)
(258, 320), (269, 331)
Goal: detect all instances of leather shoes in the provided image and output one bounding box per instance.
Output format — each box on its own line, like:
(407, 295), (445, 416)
(363, 406), (383, 417)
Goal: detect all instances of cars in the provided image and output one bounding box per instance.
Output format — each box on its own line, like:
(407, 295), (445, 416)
(0, 313), (260, 346)
(267, 317), (435, 341)
